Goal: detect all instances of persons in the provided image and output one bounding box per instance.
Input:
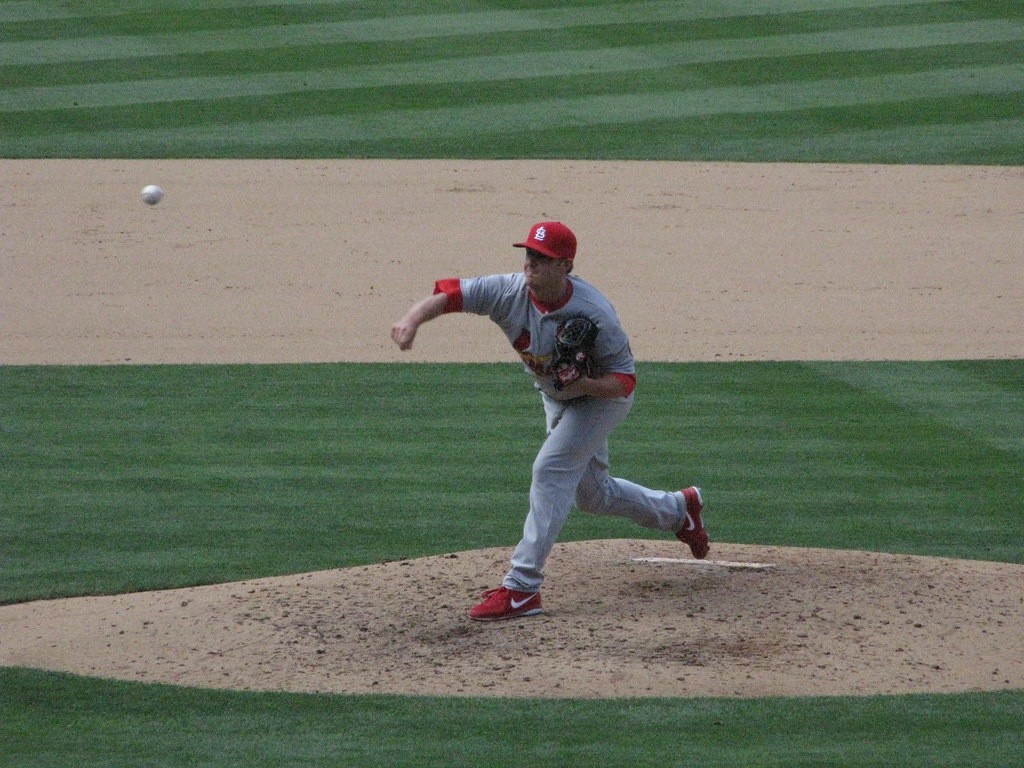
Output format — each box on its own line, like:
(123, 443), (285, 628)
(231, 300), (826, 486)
(391, 221), (710, 621)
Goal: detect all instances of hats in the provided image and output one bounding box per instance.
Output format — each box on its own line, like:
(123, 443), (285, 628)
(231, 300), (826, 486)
(511, 222), (577, 262)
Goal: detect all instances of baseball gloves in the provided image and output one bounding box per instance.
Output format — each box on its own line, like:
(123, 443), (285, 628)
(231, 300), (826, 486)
(546, 315), (599, 394)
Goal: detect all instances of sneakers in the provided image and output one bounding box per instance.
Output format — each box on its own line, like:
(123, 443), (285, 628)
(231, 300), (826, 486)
(469, 585), (543, 620)
(672, 485), (710, 560)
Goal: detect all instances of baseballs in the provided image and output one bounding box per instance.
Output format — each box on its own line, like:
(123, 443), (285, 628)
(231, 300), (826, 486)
(139, 184), (162, 205)
